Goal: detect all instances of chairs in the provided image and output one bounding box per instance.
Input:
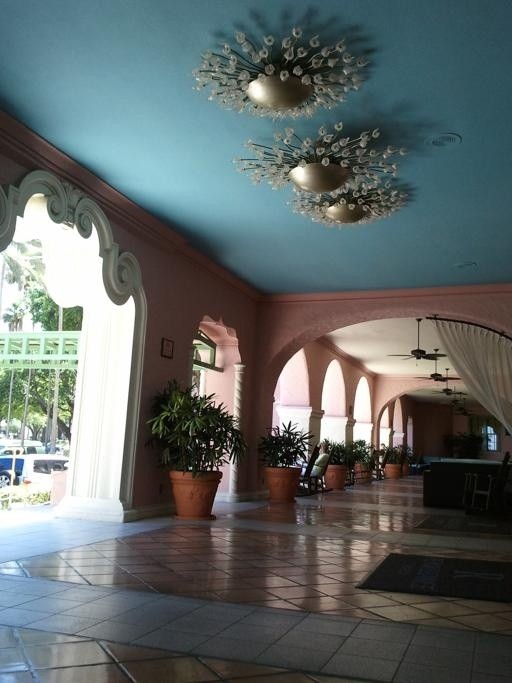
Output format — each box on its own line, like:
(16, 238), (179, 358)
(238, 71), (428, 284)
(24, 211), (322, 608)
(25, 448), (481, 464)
(463, 451), (509, 510)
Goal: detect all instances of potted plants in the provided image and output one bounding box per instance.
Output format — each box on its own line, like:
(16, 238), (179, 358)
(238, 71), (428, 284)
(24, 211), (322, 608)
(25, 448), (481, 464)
(257, 420), (316, 504)
(321, 438), (416, 492)
(143, 380), (248, 520)
(442, 431), (484, 458)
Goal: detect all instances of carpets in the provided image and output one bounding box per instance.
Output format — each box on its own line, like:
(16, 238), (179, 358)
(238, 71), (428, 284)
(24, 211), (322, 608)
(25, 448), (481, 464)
(355, 551), (512, 605)
(413, 517), (511, 536)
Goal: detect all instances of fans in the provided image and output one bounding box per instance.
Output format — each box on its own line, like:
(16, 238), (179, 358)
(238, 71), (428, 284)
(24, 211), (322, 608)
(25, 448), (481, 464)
(414, 348), (462, 385)
(432, 367), (476, 417)
(387, 318), (447, 360)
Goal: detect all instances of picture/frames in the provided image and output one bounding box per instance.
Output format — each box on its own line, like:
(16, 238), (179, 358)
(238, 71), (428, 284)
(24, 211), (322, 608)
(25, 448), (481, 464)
(161, 337), (175, 359)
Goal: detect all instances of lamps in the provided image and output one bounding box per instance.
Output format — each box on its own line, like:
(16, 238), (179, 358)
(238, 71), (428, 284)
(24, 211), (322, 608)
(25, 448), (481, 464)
(231, 118), (406, 199)
(190, 25), (369, 121)
(286, 179), (411, 226)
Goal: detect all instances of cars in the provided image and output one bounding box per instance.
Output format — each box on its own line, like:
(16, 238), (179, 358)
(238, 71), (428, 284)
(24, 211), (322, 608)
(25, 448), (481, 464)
(1, 433), (69, 488)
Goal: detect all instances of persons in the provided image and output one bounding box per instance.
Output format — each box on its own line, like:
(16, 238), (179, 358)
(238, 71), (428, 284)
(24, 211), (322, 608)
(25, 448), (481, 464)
(379, 443), (385, 468)
(293, 444), (330, 476)
